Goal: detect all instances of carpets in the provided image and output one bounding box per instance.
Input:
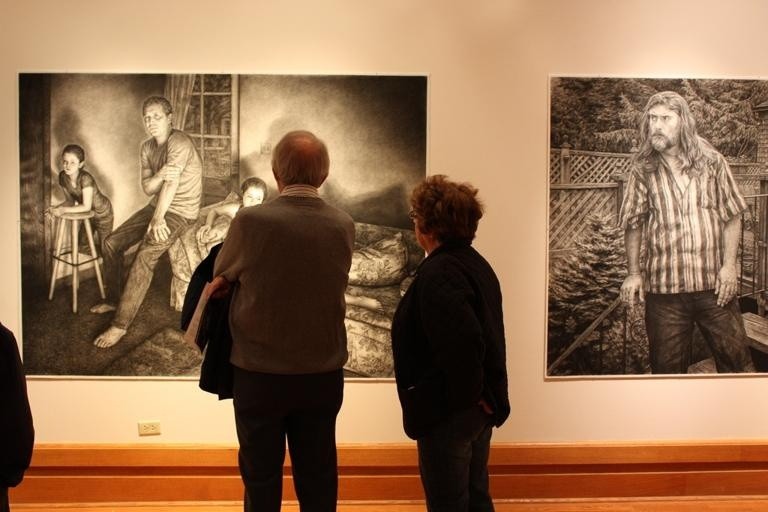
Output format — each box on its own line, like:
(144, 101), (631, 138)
(104, 318), (203, 375)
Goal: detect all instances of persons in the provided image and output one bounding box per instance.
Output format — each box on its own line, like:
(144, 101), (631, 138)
(206, 130), (356, 512)
(196, 177), (268, 241)
(90, 96), (203, 349)
(619, 91), (751, 374)
(0, 321), (36, 511)
(43, 144), (114, 259)
(391, 173), (511, 511)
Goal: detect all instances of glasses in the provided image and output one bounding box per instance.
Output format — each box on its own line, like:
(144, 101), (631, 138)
(408, 209), (416, 219)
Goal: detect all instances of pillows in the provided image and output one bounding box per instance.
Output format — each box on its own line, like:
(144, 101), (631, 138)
(349, 232), (411, 287)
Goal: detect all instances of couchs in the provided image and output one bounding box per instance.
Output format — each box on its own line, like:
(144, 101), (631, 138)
(166, 197), (421, 377)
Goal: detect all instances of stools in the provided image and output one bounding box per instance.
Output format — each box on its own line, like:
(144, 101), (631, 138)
(47, 209), (106, 313)
